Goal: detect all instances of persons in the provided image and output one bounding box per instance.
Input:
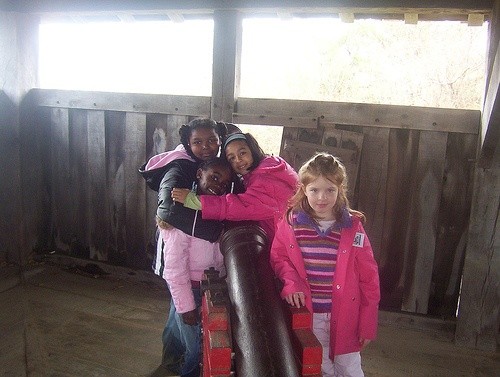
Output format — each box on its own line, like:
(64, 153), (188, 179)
(171, 132), (301, 267)
(138, 117), (234, 377)
(269, 153), (380, 377)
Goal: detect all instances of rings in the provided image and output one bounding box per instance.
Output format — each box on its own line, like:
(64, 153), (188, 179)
(173, 198), (175, 201)
(299, 293), (304, 296)
